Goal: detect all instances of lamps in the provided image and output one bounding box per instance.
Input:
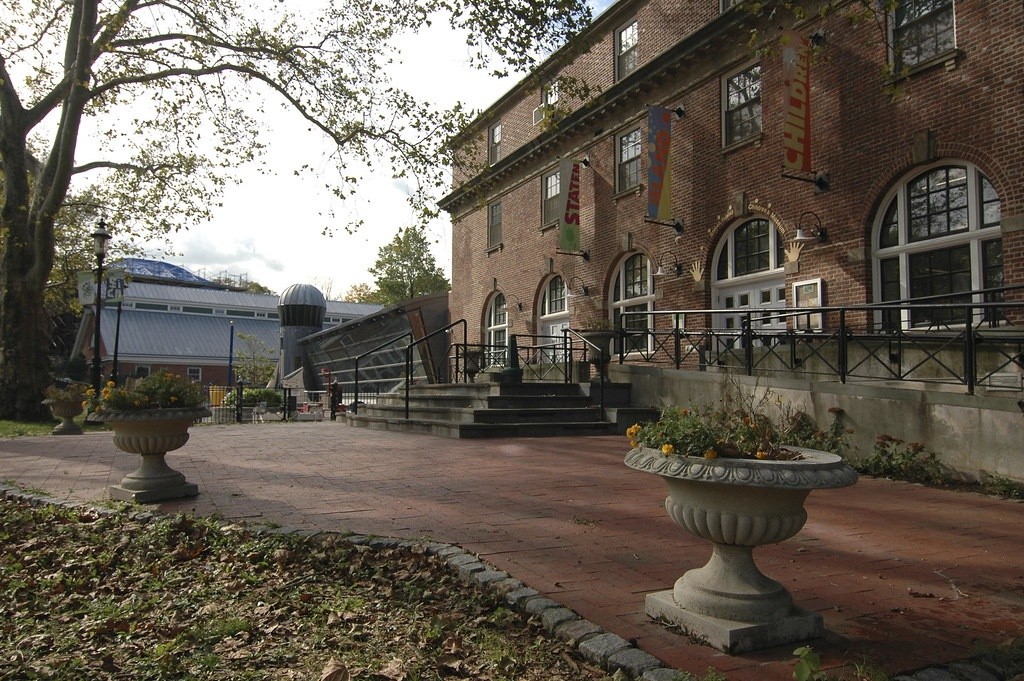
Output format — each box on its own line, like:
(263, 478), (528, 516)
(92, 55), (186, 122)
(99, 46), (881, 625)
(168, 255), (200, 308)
(648, 251), (683, 278)
(784, 211), (828, 244)
(563, 276), (589, 298)
(496, 294), (522, 312)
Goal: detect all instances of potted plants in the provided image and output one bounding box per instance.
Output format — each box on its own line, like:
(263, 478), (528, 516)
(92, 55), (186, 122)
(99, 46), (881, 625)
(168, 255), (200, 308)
(460, 346), (483, 368)
(578, 318), (619, 363)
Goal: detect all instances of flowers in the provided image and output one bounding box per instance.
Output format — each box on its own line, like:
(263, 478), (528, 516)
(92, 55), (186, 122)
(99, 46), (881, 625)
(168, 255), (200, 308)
(93, 369), (209, 415)
(624, 396), (805, 464)
(45, 382), (93, 402)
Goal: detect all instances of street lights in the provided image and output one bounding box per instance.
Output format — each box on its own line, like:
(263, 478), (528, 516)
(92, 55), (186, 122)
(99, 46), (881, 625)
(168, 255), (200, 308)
(84, 218), (113, 425)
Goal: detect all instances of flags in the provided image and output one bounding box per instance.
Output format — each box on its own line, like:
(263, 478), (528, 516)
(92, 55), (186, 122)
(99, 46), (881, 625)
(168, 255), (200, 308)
(779, 27), (814, 173)
(646, 104), (674, 221)
(559, 157), (581, 251)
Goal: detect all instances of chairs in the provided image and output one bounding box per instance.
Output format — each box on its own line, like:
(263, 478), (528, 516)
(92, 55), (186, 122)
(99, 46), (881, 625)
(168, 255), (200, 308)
(252, 402), (268, 431)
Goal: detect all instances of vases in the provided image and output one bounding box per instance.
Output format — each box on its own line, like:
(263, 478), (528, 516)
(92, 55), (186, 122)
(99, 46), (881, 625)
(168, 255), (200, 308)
(40, 399), (90, 434)
(624, 446), (861, 656)
(82, 407), (214, 506)
